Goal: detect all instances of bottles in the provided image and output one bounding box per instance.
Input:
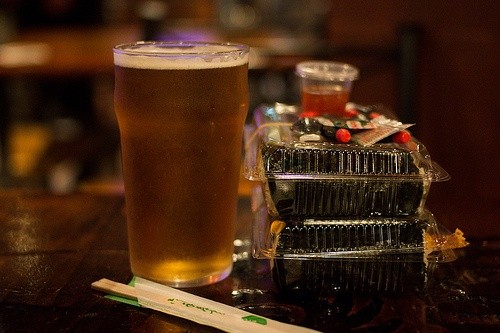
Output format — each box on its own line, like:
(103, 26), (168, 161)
(380, 130), (411, 143)
(321, 126), (351, 143)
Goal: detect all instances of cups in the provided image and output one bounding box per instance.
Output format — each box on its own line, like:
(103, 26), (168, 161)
(294, 61), (358, 116)
(113, 37), (250, 289)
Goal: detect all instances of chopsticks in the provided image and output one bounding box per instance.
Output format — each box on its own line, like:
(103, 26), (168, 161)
(91, 278), (136, 300)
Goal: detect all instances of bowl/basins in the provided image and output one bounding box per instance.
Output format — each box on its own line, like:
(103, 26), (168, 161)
(250, 208), (457, 294)
(242, 122), (450, 216)
